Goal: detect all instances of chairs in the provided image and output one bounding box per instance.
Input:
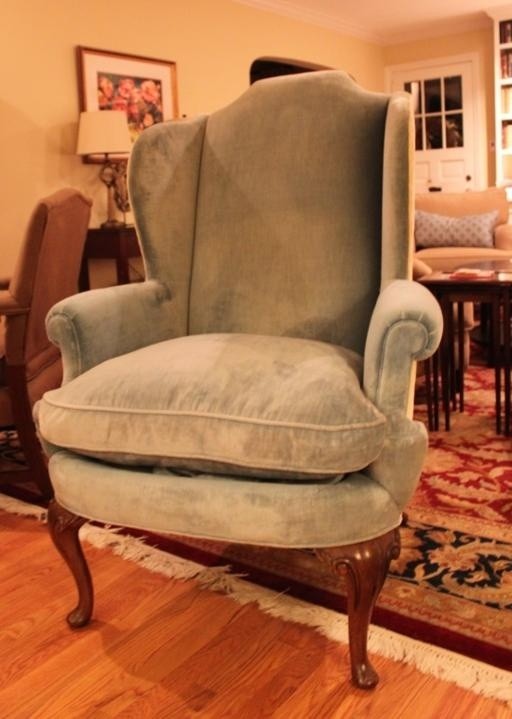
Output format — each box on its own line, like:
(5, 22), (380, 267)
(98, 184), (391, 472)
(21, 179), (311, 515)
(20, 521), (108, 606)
(0, 71), (512, 689)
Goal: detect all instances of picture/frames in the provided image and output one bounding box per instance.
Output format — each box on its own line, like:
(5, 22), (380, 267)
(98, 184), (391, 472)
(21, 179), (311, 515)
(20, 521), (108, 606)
(75, 45), (180, 162)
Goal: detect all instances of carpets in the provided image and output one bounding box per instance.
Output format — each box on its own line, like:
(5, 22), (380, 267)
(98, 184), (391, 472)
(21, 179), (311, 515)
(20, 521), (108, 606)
(0, 354), (512, 709)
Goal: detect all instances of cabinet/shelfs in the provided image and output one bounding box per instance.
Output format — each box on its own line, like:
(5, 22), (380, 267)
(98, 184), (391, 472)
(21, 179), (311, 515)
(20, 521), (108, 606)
(492, 18), (512, 202)
